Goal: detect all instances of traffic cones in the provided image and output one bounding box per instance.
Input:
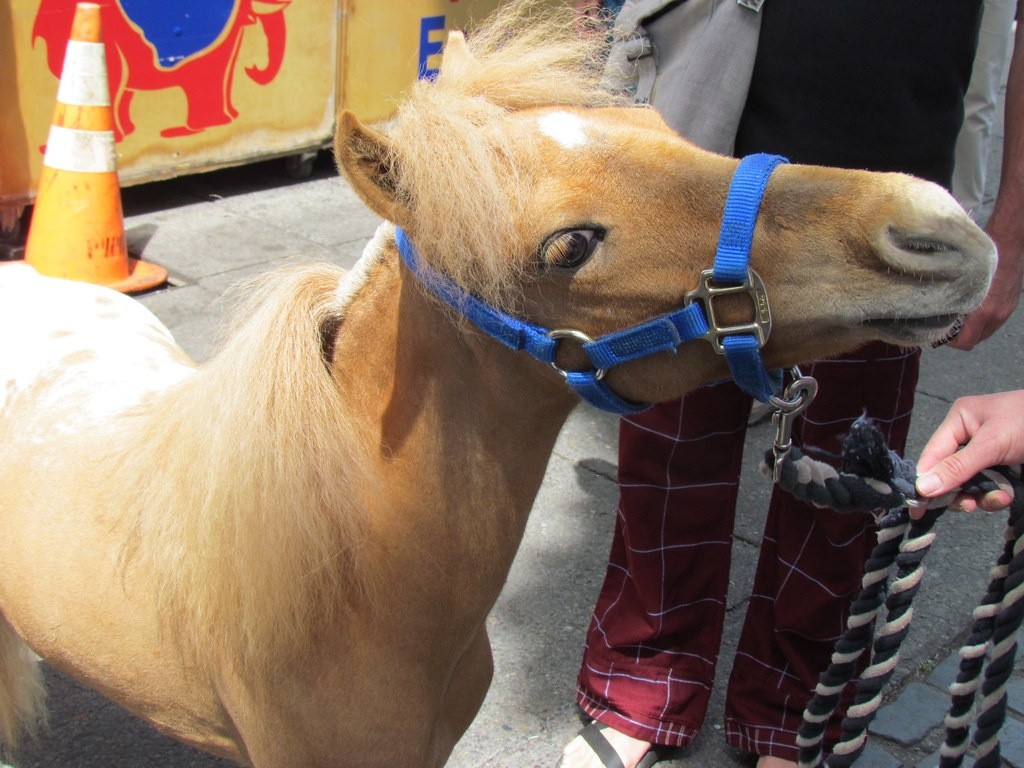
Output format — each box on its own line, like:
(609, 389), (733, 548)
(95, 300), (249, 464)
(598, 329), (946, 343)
(22, 3), (167, 294)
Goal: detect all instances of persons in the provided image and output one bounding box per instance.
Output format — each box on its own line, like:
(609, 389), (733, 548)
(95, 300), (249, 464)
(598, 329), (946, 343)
(564, 0), (1022, 768)
(912, 385), (1024, 523)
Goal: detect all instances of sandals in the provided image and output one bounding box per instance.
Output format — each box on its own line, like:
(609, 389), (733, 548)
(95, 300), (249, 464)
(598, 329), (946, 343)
(554, 718), (673, 768)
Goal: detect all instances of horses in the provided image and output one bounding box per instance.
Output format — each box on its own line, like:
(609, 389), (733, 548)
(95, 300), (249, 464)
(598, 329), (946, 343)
(1, 0), (1000, 768)
